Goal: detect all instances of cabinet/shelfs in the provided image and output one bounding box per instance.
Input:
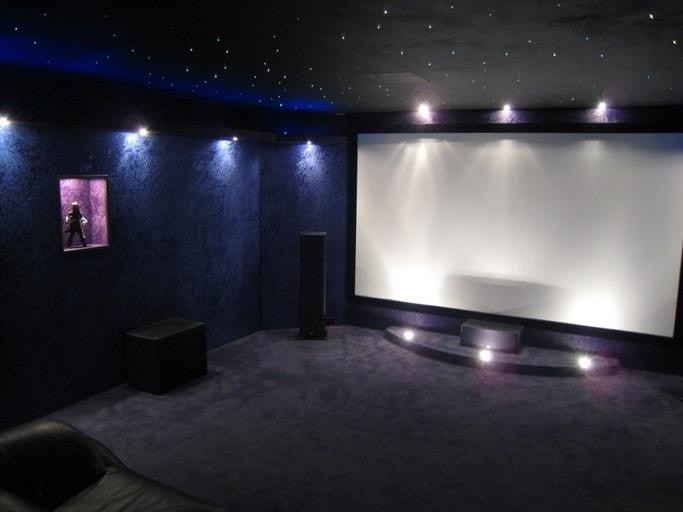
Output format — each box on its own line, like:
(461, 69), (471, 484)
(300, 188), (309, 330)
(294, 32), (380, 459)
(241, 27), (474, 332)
(123, 315), (208, 395)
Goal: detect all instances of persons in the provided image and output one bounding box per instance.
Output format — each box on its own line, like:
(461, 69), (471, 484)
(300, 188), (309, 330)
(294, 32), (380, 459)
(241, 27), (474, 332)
(64, 201), (88, 248)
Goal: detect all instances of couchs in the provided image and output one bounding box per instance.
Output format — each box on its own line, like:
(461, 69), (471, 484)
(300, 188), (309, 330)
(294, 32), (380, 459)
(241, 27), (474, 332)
(0, 416), (232, 511)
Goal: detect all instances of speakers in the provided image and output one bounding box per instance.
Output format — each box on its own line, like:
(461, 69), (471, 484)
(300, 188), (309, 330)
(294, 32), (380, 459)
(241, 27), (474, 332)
(302, 230), (328, 340)
